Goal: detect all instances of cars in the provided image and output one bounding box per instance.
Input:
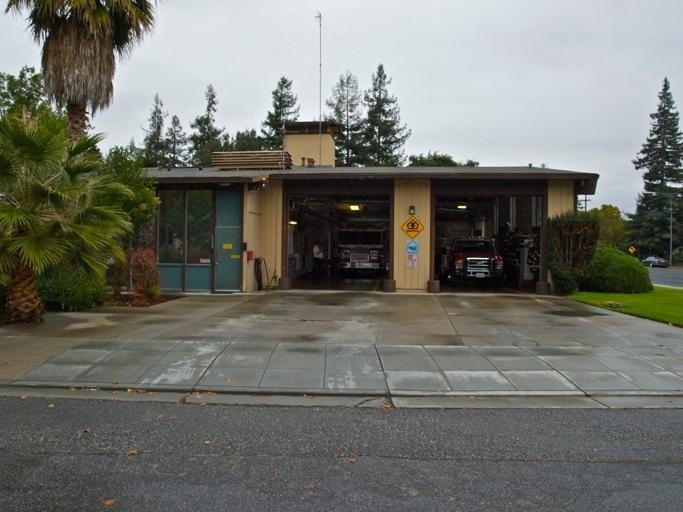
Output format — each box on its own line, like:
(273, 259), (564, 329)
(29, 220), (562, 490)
(640, 253), (669, 270)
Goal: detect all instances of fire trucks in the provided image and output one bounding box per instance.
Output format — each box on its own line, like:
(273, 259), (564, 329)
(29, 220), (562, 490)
(336, 226), (387, 271)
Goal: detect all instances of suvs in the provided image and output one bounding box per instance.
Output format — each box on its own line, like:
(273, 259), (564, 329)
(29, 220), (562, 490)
(449, 236), (504, 285)
